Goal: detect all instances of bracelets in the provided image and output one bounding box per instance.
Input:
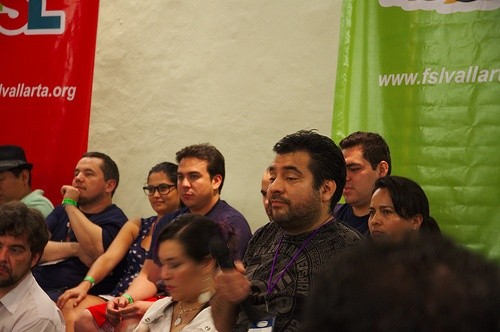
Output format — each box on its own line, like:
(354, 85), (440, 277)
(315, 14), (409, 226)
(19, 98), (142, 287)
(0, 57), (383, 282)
(62, 198), (78, 207)
(122, 293), (134, 304)
(84, 276), (95, 288)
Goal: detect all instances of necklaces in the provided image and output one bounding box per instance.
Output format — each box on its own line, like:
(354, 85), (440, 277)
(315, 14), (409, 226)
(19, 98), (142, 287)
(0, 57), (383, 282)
(173, 299), (209, 327)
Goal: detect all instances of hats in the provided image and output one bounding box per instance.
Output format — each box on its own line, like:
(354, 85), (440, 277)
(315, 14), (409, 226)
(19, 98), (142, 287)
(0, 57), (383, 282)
(0, 145), (33, 171)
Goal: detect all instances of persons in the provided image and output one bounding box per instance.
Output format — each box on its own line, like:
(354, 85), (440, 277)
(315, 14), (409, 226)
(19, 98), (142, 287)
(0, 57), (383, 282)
(0, 130), (442, 332)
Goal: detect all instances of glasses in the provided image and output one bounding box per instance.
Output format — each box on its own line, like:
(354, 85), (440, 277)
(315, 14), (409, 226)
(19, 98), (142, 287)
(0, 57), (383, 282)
(143, 184), (177, 196)
